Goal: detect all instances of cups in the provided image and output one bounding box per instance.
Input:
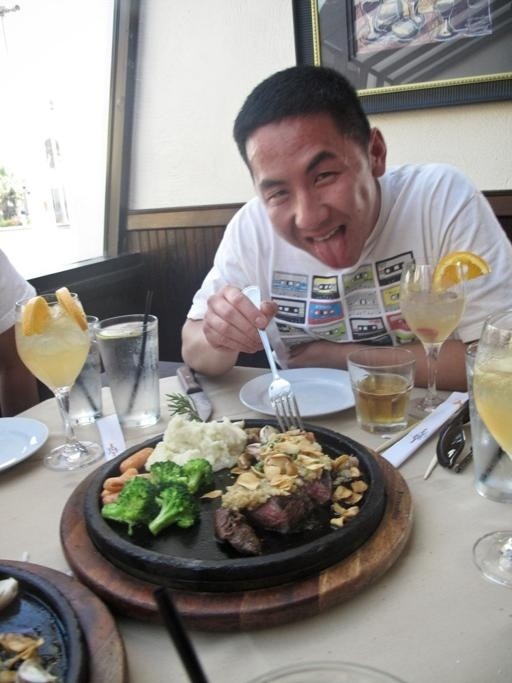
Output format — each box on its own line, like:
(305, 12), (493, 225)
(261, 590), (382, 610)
(345, 344), (417, 434)
(51, 315), (107, 426)
(96, 312), (167, 433)
(463, 338), (511, 507)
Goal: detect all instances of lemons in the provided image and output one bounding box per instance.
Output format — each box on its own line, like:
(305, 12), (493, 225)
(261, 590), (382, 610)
(56, 287), (89, 331)
(22, 296), (52, 336)
(430, 252), (492, 294)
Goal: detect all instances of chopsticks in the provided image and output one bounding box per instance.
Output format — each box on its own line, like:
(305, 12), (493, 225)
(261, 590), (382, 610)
(370, 400), (461, 484)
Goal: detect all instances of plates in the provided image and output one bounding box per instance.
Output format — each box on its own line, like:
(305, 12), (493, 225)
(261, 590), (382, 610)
(238, 363), (361, 416)
(243, 661), (398, 682)
(0, 415), (51, 473)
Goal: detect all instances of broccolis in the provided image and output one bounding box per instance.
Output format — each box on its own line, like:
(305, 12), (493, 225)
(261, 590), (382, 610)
(101, 458), (213, 536)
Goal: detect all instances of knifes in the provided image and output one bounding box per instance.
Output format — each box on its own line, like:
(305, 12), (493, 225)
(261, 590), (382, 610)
(176, 363), (214, 426)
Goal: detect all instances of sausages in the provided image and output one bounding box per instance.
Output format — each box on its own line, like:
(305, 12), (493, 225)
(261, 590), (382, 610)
(100, 446), (155, 505)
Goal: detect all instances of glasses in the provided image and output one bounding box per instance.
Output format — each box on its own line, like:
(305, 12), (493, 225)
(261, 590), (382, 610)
(437, 399), (473, 473)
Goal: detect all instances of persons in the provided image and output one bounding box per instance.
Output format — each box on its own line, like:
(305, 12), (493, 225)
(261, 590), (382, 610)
(180, 65), (512, 392)
(0, 248), (40, 417)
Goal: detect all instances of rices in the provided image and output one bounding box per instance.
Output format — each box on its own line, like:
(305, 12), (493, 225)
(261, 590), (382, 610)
(144, 416), (247, 471)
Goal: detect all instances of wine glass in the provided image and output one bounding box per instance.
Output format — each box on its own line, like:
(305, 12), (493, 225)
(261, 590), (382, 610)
(13, 289), (105, 474)
(470, 309), (512, 592)
(397, 255), (466, 420)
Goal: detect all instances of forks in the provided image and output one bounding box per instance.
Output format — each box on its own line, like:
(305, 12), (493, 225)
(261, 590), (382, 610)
(241, 284), (308, 436)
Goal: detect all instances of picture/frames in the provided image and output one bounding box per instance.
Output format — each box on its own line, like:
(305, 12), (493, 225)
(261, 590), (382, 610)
(291, 0), (511, 116)
